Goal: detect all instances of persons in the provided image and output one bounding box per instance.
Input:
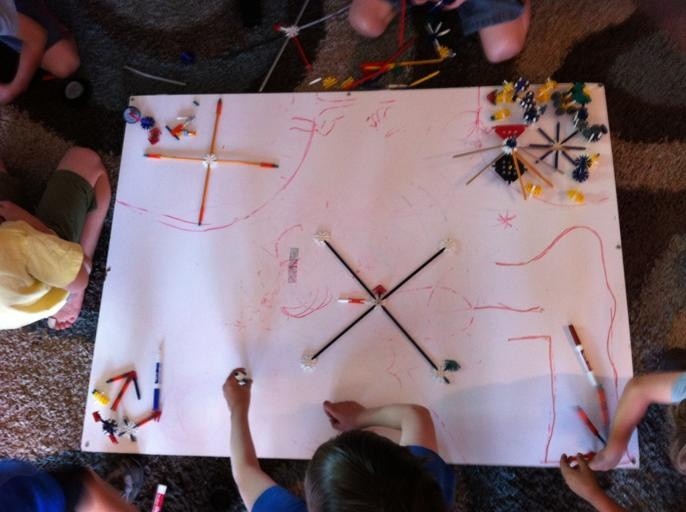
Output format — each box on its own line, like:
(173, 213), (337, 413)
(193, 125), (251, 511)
(0, 451), (147, 511)
(0, 146), (111, 334)
(219, 367), (453, 512)
(558, 371), (685, 511)
(0, 1), (82, 108)
(343, 0), (533, 62)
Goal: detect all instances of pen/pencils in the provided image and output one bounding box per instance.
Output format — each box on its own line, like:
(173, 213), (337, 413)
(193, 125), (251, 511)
(596, 385), (609, 442)
(152, 484), (167, 512)
(568, 325), (601, 390)
(570, 452), (596, 460)
(153, 362), (160, 411)
(232, 370), (249, 384)
(574, 405), (606, 447)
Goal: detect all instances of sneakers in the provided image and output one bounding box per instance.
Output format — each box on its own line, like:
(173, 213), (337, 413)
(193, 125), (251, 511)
(659, 348), (685, 371)
(89, 455), (143, 504)
